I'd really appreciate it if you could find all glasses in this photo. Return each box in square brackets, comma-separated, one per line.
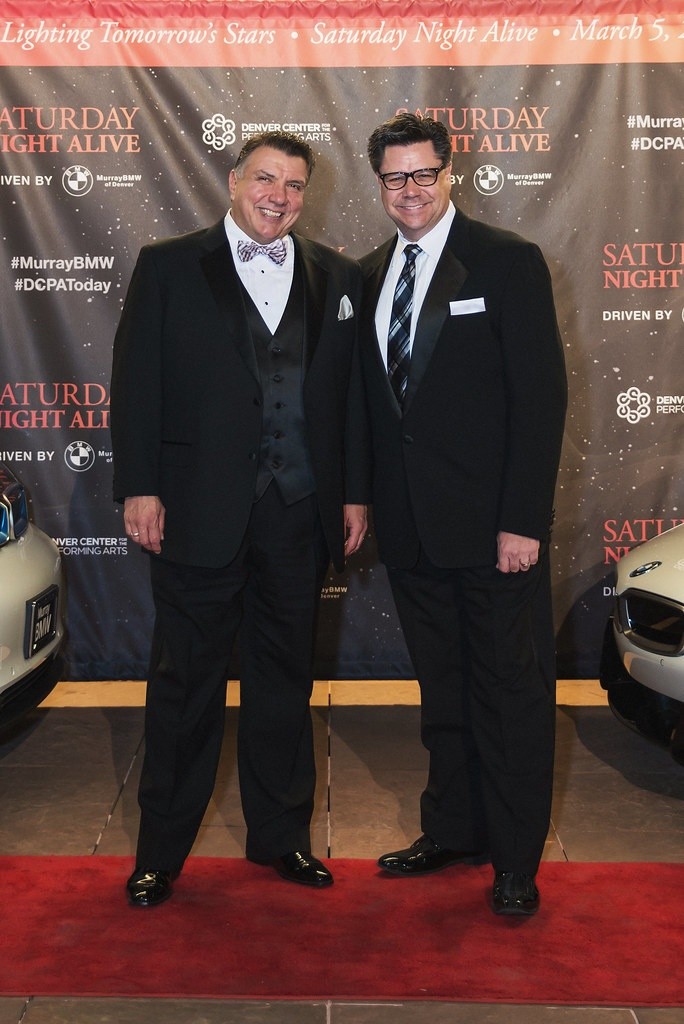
[376, 164, 446, 190]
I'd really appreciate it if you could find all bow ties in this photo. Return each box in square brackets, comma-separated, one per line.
[237, 239, 285, 269]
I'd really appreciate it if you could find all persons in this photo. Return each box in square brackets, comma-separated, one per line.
[355, 113, 568, 914]
[108, 132, 373, 907]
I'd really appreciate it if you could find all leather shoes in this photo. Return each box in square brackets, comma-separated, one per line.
[377, 834, 492, 875]
[491, 870, 540, 915]
[246, 850, 333, 887]
[126, 865, 181, 906]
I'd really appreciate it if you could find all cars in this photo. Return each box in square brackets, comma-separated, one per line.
[602, 520, 684, 704]
[1, 463, 70, 728]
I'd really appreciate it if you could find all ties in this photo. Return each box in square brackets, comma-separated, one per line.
[387, 244, 423, 412]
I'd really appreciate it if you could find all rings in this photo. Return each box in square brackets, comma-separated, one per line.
[131, 532, 139, 536]
[521, 562, 530, 567]
